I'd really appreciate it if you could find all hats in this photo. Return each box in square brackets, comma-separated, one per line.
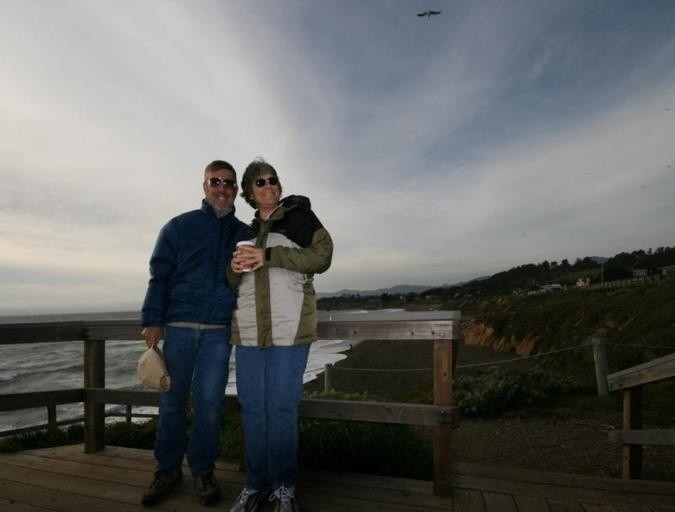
[137, 343, 172, 393]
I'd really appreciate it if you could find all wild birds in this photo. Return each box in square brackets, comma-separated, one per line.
[417, 9, 442, 18]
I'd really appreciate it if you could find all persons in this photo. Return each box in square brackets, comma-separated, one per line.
[226, 163, 330, 511]
[141, 162, 249, 507]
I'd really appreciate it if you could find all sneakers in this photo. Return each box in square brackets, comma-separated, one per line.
[229, 485, 272, 512]
[141, 467, 182, 506]
[194, 465, 222, 506]
[271, 479, 301, 512]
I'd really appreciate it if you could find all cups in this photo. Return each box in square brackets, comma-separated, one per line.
[235, 241, 254, 273]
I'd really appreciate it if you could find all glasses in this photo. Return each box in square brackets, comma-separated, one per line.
[206, 177, 236, 190]
[252, 176, 278, 187]
